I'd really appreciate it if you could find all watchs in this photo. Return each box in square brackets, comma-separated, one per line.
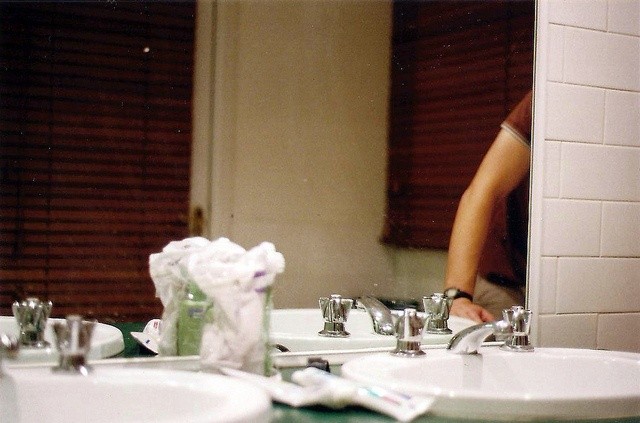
[443, 287, 474, 302]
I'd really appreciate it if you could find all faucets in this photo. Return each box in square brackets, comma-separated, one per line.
[447, 305, 535, 354]
[355, 294, 396, 335]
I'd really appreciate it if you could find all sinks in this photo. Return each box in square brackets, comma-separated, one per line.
[269, 307, 481, 352]
[1, 315, 125, 362]
[341, 343, 640, 420]
[2, 366, 273, 423]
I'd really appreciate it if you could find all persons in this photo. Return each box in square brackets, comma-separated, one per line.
[443, 89, 532, 324]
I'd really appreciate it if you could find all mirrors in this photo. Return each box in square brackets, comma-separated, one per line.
[1, 0, 537, 365]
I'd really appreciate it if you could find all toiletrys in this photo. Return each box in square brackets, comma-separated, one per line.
[242, 285, 272, 376]
